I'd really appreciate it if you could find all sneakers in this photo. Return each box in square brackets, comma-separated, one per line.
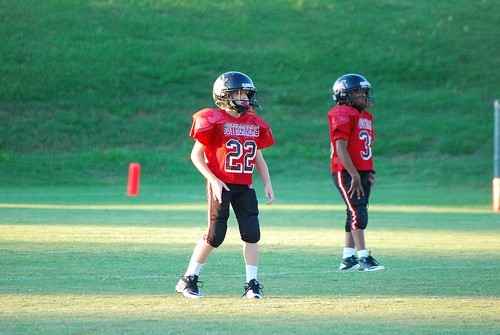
[339, 255, 385, 271]
[176, 274, 203, 299]
[244, 278, 264, 298]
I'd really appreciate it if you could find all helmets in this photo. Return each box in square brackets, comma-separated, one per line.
[213, 71, 258, 114]
[333, 73, 373, 107]
